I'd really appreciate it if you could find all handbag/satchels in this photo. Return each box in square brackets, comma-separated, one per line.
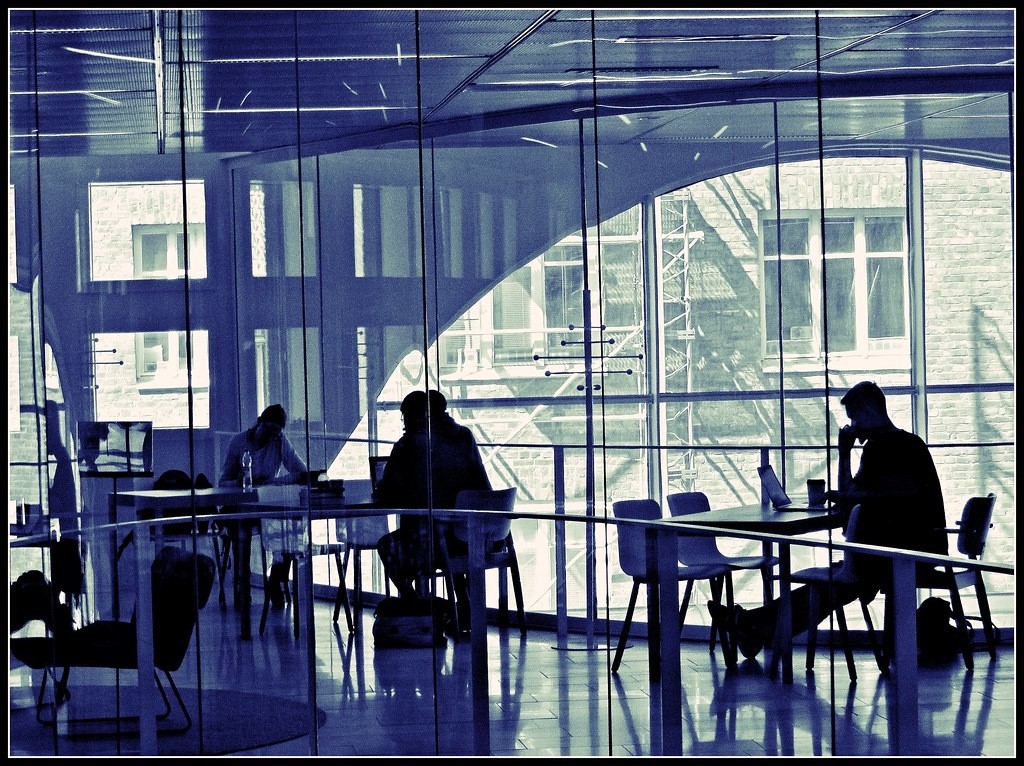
[373, 596, 449, 649]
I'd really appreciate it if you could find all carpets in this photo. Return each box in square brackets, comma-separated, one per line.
[9, 684, 329, 757]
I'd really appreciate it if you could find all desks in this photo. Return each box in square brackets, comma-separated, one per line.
[108, 480, 386, 641]
[644, 495, 853, 687]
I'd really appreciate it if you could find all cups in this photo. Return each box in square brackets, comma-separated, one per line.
[806, 478, 825, 505]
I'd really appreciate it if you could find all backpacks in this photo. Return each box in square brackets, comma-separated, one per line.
[917, 597, 1001, 658]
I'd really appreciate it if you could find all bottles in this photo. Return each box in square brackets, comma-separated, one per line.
[241, 449, 253, 488]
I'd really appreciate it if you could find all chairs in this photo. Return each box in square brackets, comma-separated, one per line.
[9, 453, 529, 743]
[609, 493, 999, 680]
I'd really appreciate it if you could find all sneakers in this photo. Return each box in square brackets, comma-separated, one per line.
[708, 601, 763, 659]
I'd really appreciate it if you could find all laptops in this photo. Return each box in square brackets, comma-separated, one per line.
[369, 455, 390, 494]
[757, 464, 834, 511]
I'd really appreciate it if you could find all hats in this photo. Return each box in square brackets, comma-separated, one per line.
[261, 404, 287, 429]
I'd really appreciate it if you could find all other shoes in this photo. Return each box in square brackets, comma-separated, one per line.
[237, 587, 252, 603]
[268, 577, 284, 604]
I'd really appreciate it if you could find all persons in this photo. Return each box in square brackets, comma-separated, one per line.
[217, 404, 313, 610]
[372, 390, 492, 644]
[708, 381, 948, 659]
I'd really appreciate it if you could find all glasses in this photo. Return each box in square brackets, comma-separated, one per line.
[401, 414, 404, 421]
